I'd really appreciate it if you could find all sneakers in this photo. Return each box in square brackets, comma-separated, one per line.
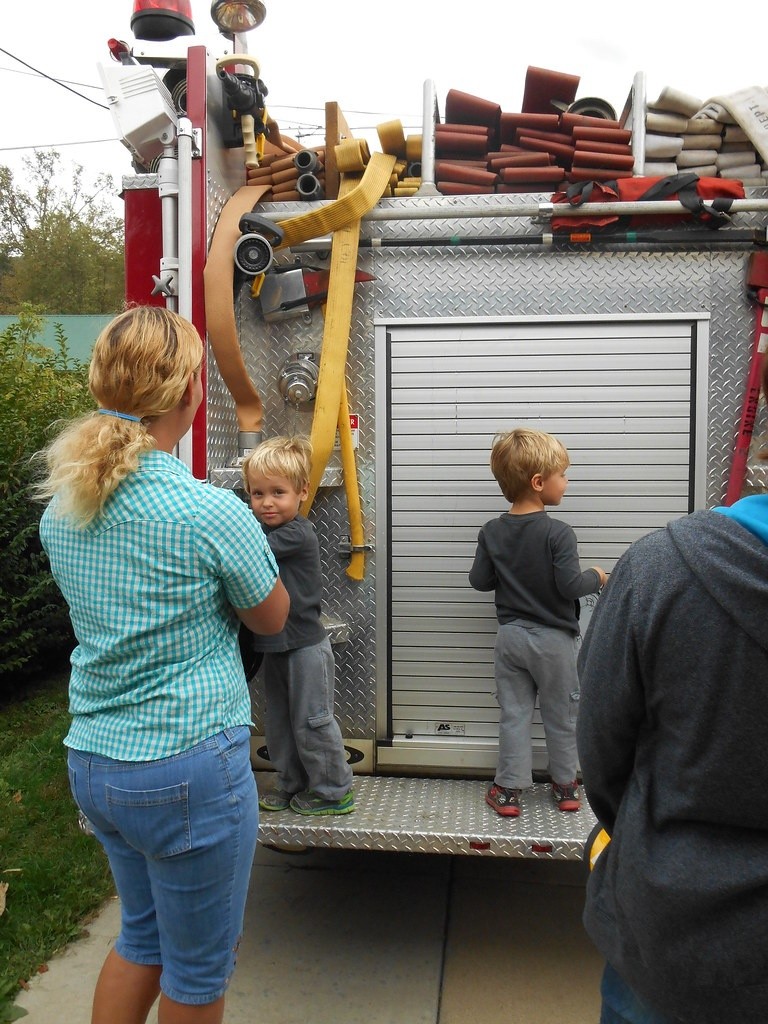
[258, 789, 294, 811]
[485, 783, 522, 817]
[551, 778, 581, 811]
[289, 790, 354, 816]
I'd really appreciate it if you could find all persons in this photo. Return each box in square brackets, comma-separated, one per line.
[31, 306, 292, 1022]
[467, 429, 608, 817]
[574, 493, 768, 1024]
[246, 435, 357, 817]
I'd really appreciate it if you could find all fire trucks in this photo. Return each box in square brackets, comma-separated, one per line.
[104, 0, 767, 874]
